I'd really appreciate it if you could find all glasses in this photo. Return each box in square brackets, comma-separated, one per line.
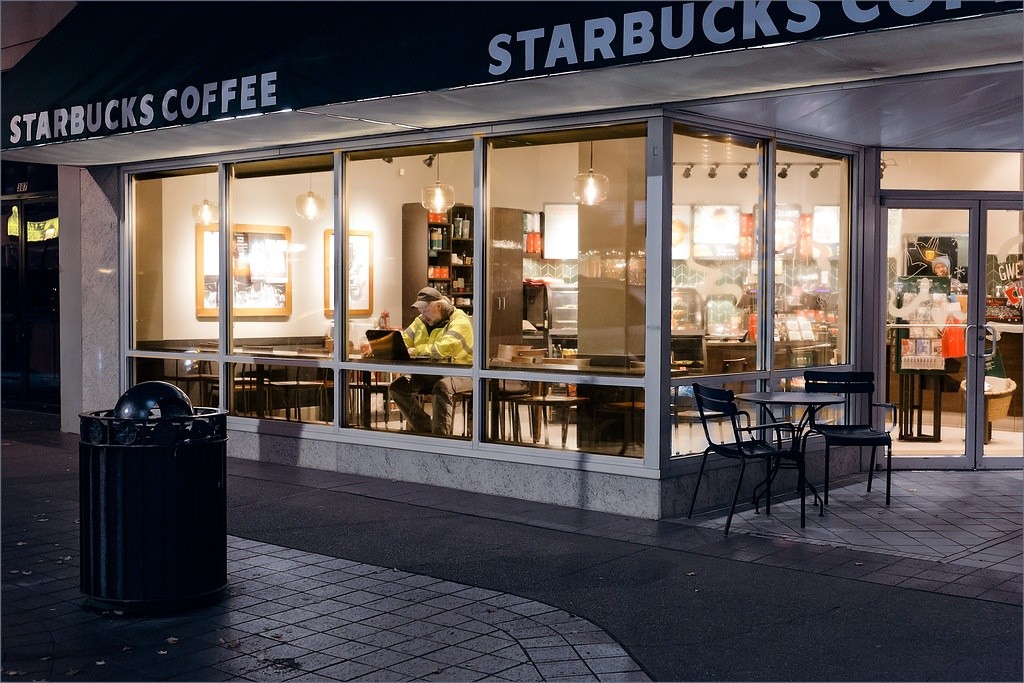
[415, 292, 442, 301]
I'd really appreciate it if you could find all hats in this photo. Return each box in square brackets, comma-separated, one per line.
[932, 258, 950, 274]
[411, 287, 442, 307]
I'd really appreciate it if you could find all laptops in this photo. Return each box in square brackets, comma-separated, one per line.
[365, 330, 431, 360]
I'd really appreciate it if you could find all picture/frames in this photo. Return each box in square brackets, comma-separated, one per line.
[194, 223, 292, 318]
[324, 228, 374, 317]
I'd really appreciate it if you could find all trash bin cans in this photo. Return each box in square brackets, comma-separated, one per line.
[78, 381, 230, 615]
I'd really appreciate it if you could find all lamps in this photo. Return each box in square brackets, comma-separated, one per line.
[296, 173, 324, 220]
[193, 171, 218, 226]
[421, 152, 456, 213]
[571, 139, 609, 208]
[382, 152, 437, 168]
[673, 161, 841, 178]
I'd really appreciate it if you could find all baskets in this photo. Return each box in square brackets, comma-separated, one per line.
[960, 375, 1018, 421]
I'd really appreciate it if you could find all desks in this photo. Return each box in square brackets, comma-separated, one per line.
[733, 391, 846, 519]
[153, 349, 644, 457]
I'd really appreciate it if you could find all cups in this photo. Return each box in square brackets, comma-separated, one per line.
[360, 336, 370, 353]
[464, 298, 471, 306]
[462, 220, 470, 238]
[454, 217, 463, 238]
[452, 297, 455, 305]
[455, 297, 466, 305]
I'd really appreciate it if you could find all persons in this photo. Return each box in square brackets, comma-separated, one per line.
[931, 256, 962, 295]
[389, 286, 473, 436]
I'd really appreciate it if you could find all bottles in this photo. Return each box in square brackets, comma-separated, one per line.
[428, 282, 448, 295]
[562, 348, 578, 359]
[428, 265, 449, 279]
[428, 226, 448, 250]
[551, 343, 563, 358]
[1005, 286, 1009, 297]
[995, 286, 1003, 297]
[523, 227, 541, 253]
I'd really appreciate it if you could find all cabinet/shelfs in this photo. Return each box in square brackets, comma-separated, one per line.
[401, 204, 474, 334]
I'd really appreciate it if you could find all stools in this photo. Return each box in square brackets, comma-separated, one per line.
[895, 355, 946, 441]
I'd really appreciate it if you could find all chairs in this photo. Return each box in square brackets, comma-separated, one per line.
[796, 370, 899, 509]
[149, 342, 748, 457]
[688, 382, 806, 539]
